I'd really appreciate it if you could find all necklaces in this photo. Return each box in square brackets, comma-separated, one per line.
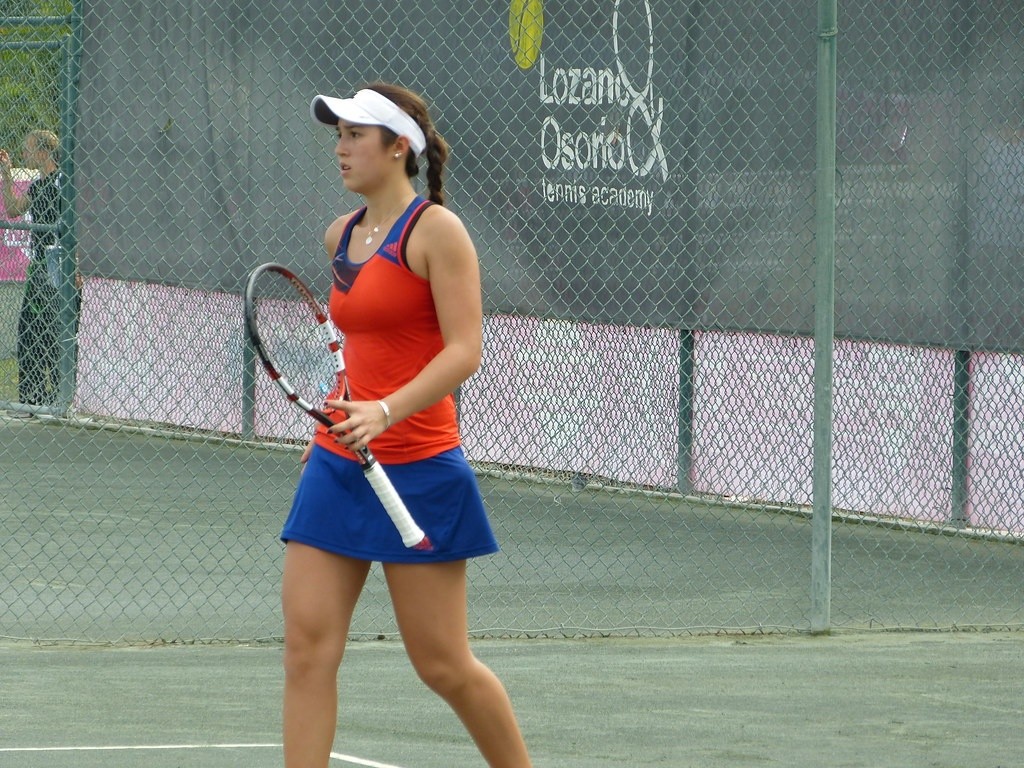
[366, 192, 415, 245]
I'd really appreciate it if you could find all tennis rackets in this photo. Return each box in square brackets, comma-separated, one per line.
[241, 263, 431, 551]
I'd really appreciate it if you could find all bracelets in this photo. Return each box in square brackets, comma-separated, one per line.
[377, 400, 391, 431]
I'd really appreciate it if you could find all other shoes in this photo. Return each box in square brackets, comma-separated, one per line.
[6, 410, 32, 418]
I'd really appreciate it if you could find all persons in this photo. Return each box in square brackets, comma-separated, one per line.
[0, 129, 61, 418]
[279, 81, 532, 768]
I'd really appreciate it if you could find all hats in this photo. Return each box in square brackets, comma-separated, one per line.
[309, 89, 426, 158]
